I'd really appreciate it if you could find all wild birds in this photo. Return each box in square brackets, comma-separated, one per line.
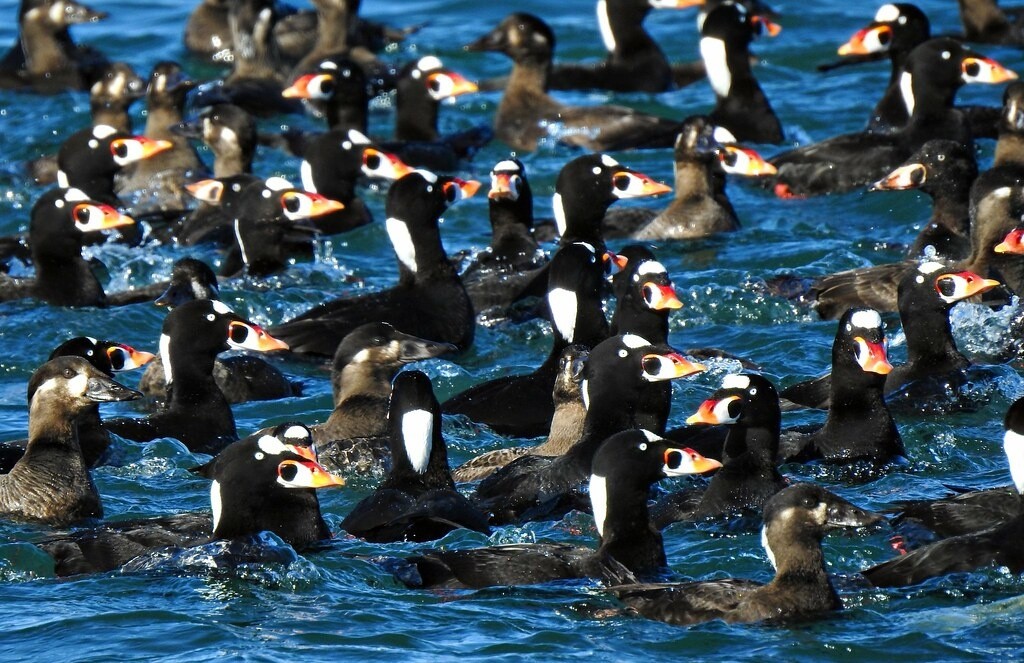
[1, 0, 1024, 632]
